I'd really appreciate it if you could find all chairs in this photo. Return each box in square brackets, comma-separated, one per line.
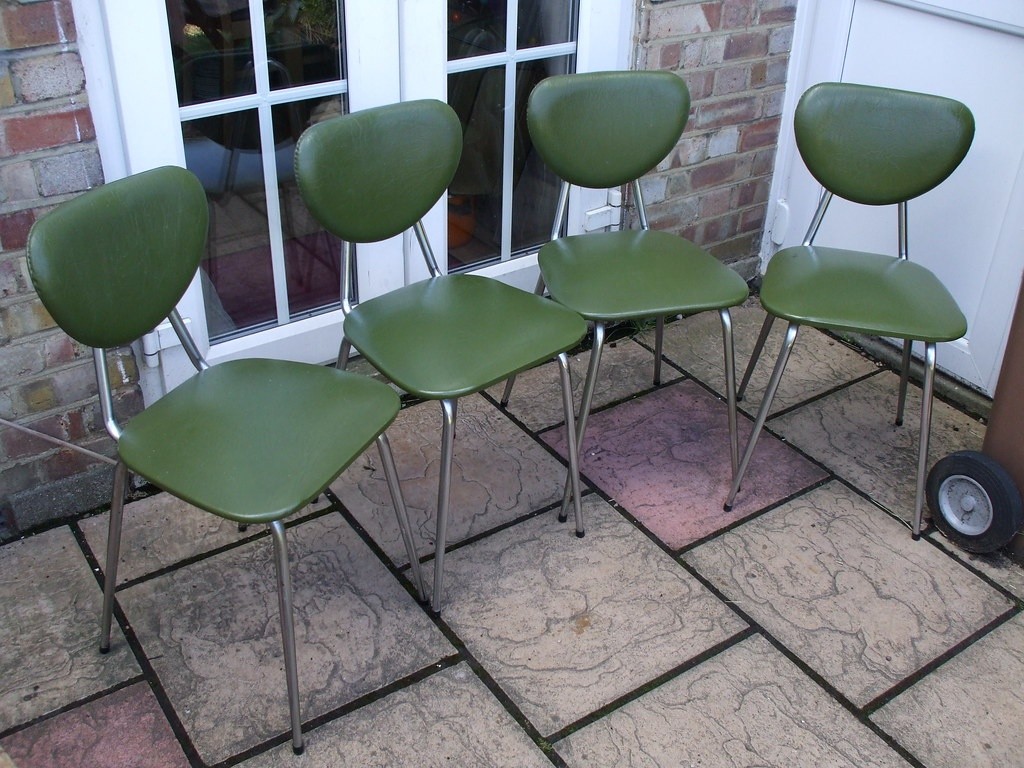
[722, 82, 977, 540]
[27, 167, 431, 755]
[295, 98, 585, 616]
[496, 69, 752, 525]
[176, 0, 546, 311]
